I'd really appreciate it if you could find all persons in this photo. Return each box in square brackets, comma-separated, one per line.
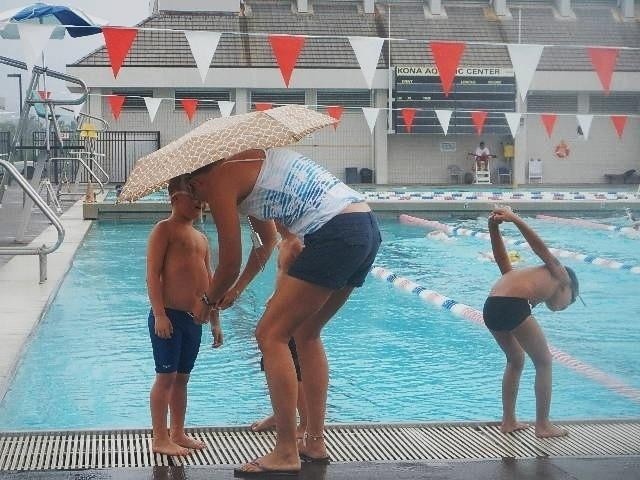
[483, 205, 580, 439]
[184, 145, 383, 474]
[245, 217, 309, 439]
[472, 141, 493, 170]
[144, 173, 224, 456]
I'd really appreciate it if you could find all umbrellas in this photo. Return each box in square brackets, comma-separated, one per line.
[0, 0, 111, 153]
[113, 101, 342, 205]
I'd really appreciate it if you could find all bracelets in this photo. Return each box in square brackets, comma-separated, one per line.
[202, 292, 218, 308]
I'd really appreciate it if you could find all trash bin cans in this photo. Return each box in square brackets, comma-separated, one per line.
[361, 167, 372, 183]
[345, 168, 358, 184]
[448, 163, 465, 184]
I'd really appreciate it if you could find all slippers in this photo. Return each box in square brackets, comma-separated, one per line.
[234, 447, 330, 480]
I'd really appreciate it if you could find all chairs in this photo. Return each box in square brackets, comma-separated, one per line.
[471, 159, 492, 184]
